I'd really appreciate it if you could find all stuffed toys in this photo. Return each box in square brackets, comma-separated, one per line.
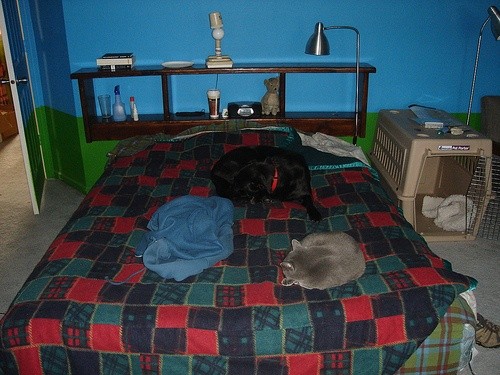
[262, 77, 280, 116]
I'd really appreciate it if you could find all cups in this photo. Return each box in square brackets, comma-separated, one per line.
[99, 96, 112, 118]
[207, 90, 221, 120]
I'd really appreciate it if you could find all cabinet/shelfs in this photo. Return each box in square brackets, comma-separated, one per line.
[70, 62, 376, 139]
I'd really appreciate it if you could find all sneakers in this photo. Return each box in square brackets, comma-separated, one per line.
[476, 312, 500, 348]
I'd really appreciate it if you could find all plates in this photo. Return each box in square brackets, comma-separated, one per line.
[162, 60, 194, 68]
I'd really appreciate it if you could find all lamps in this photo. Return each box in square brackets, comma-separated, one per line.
[204, 11, 233, 69]
[466, 5, 500, 126]
[304, 20, 361, 146]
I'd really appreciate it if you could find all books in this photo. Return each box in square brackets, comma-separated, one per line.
[96, 53, 135, 69]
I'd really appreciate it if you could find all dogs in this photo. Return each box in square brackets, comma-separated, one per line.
[208, 143, 323, 224]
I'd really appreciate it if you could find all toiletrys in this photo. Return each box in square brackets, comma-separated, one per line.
[129, 95, 136, 115]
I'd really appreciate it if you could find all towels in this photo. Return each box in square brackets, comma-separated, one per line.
[422, 192, 478, 234]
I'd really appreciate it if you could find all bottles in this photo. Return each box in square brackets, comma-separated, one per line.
[130, 97, 134, 119]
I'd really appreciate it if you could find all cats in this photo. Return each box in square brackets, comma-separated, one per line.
[279, 230, 367, 292]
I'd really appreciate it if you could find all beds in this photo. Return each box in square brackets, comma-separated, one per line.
[0, 117, 477, 375]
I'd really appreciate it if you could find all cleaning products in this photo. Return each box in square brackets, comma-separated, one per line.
[113, 84, 127, 121]
